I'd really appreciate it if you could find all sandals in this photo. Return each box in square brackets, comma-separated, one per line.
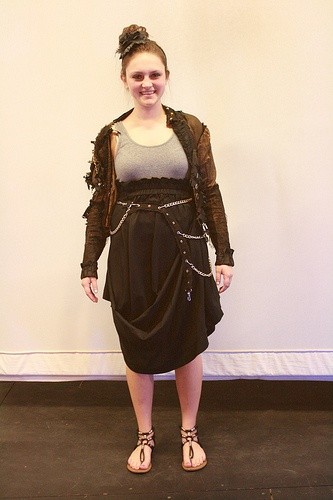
[180, 425, 207, 472]
[126, 426, 156, 475]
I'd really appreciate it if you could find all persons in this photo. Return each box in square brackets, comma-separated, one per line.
[79, 24, 234, 476]
